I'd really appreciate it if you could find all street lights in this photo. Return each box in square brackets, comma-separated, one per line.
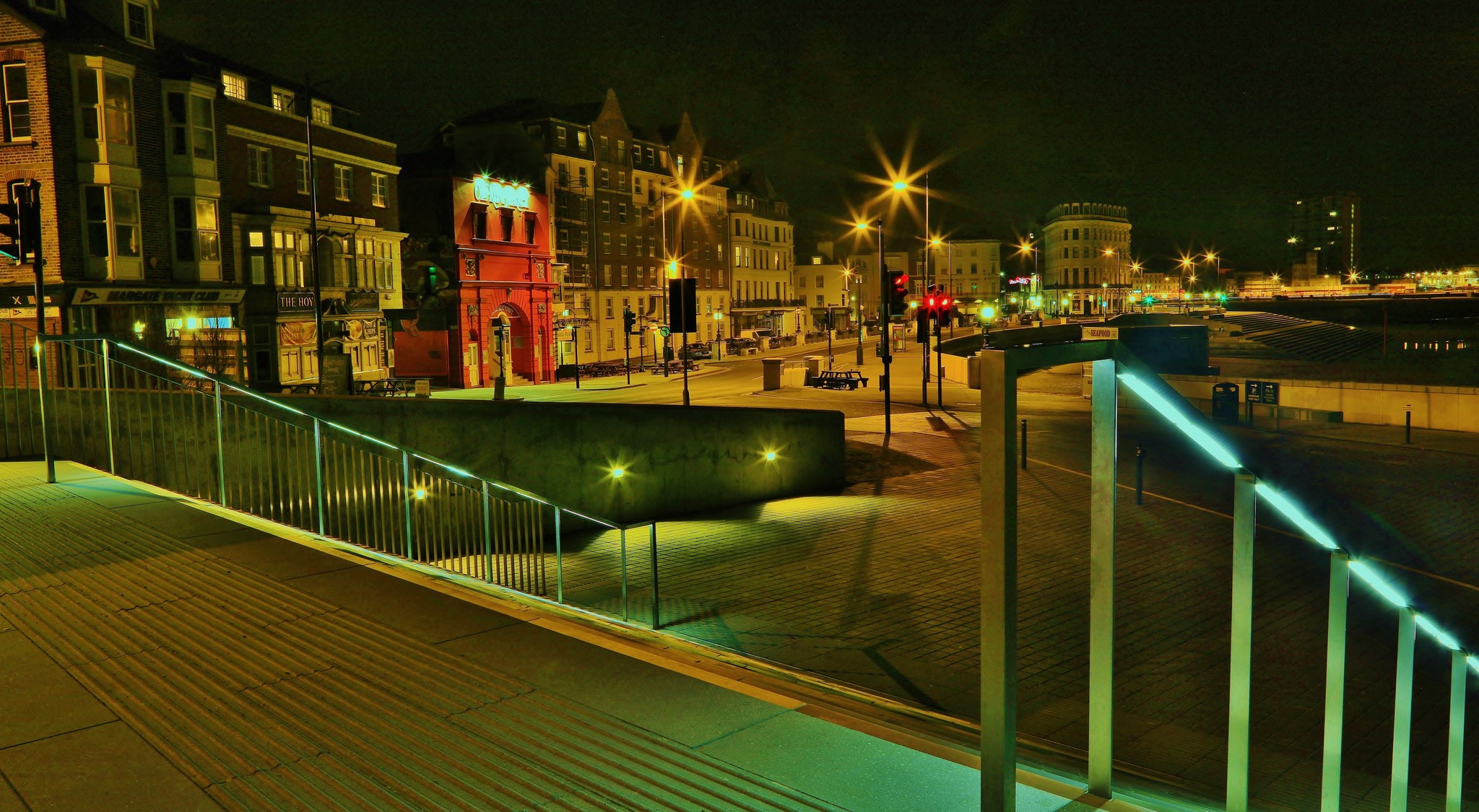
[678, 188, 695, 407]
[1208, 255, 1219, 300]
[713, 308, 723, 361]
[932, 239, 958, 339]
[1106, 249, 1121, 314]
[892, 179, 954, 339]
[1191, 277, 1195, 310]
[795, 304, 803, 333]
[1133, 264, 1143, 311]
[1022, 246, 1038, 314]
[567, 326, 581, 389]
[499, 324, 512, 377]
[1178, 258, 1188, 313]
[1165, 277, 1170, 300]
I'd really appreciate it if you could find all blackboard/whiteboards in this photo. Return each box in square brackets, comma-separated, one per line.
[320, 355, 355, 396]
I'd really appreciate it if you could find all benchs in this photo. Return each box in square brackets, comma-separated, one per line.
[579, 368, 611, 378]
[649, 363, 700, 375]
[812, 376, 869, 390]
[616, 366, 640, 376]
[355, 386, 437, 396]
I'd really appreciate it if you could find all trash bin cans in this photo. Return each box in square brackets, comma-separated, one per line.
[668, 347, 674, 362]
[876, 342, 882, 357]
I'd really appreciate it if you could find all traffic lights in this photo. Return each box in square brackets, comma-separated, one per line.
[0, 198, 29, 267]
[926, 294, 937, 319]
[628, 311, 636, 327]
[888, 269, 909, 316]
[937, 295, 951, 328]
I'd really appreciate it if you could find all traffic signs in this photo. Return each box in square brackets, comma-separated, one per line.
[830, 304, 839, 306]
[1244, 380, 1279, 405]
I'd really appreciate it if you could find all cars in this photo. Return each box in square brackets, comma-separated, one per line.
[983, 315, 1010, 329]
[1020, 314, 1042, 325]
[676, 327, 781, 360]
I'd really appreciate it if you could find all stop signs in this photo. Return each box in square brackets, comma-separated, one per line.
[650, 323, 658, 332]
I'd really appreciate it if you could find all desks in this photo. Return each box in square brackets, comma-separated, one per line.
[816, 371, 862, 390]
[281, 380, 410, 397]
[578, 361, 636, 373]
[654, 359, 697, 375]
[382, 378, 434, 397]
